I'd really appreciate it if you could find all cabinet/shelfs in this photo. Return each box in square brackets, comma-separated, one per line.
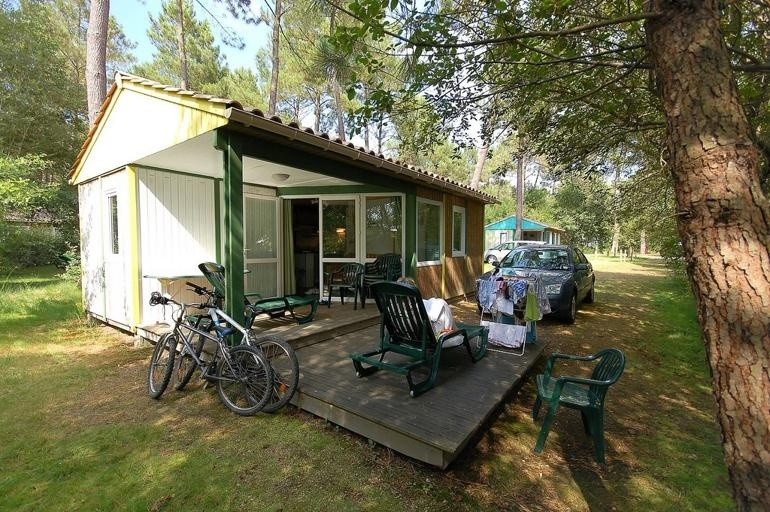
[472, 269, 543, 356]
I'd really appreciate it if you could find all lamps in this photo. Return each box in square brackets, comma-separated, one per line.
[272, 173, 290, 182]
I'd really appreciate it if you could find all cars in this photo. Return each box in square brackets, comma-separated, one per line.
[475, 245, 595, 325]
[485, 240, 547, 266]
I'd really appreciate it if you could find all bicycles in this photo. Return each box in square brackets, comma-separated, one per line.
[146, 280, 299, 416]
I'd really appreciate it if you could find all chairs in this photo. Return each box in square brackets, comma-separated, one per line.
[532, 349, 627, 463]
[326, 251, 402, 310]
[349, 280, 491, 396]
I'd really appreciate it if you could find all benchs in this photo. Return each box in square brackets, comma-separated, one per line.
[185, 261, 320, 333]
